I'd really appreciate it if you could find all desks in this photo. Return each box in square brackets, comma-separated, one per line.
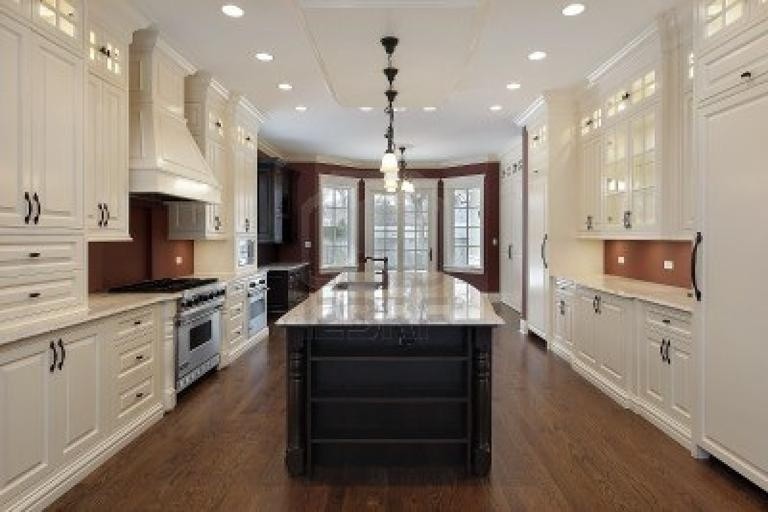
[273, 271, 508, 481]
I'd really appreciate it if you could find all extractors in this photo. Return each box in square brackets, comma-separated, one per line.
[129, 29, 220, 203]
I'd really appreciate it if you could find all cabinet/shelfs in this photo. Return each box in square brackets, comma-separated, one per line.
[691, 1, 768, 494]
[604, 33, 690, 240]
[527, 89, 548, 349]
[0, 0, 165, 512]
[568, 76, 602, 240]
[499, 126, 525, 319]
[195, 97, 258, 274]
[633, 299, 699, 458]
[267, 260, 310, 317]
[165, 293, 174, 374]
[168, 76, 231, 240]
[572, 283, 633, 410]
[548, 275, 572, 369]
[258, 169, 283, 244]
[222, 272, 269, 369]
[174, 280, 221, 373]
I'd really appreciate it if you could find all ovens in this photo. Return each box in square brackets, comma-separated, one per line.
[174, 300, 220, 407]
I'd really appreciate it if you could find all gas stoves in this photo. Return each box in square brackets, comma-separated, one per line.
[108, 277, 226, 317]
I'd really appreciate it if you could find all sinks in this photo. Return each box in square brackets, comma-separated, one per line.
[332, 282, 379, 291]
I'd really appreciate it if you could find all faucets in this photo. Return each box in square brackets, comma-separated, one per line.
[361, 255, 388, 288]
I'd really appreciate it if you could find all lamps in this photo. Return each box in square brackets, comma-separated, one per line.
[377, 34, 415, 194]
